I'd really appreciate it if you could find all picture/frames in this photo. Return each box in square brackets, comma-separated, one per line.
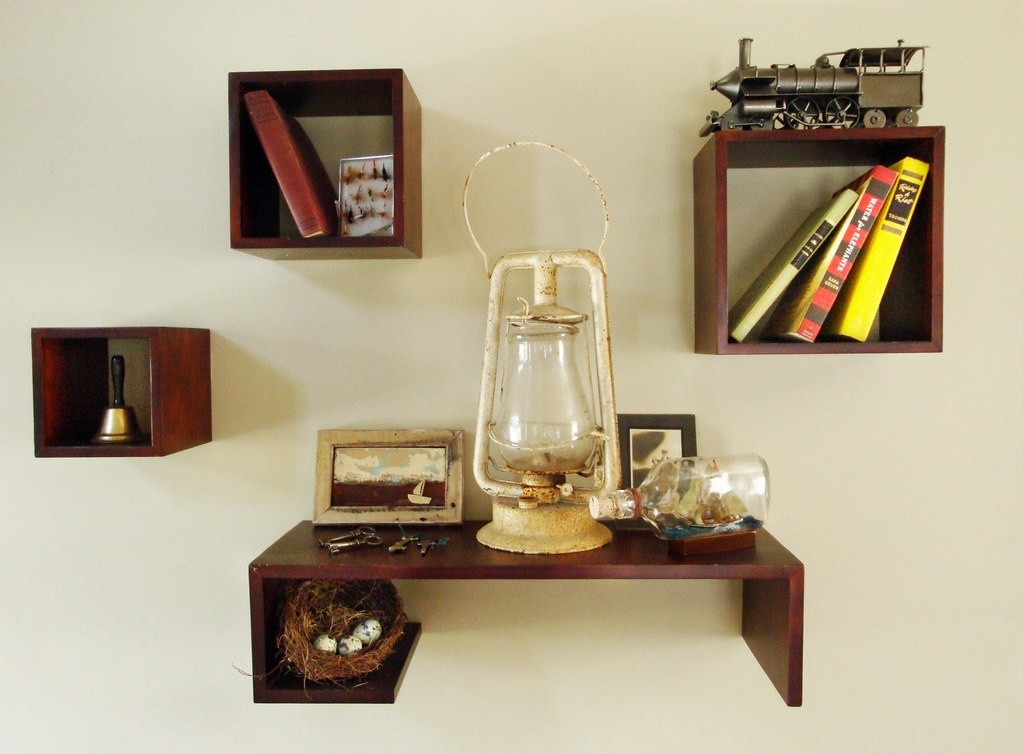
[616, 413, 698, 533]
[314, 430, 463, 527]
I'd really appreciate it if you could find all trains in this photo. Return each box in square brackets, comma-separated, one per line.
[697, 37, 929, 138]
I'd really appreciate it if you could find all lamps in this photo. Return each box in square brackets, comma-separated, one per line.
[464, 141, 623, 556]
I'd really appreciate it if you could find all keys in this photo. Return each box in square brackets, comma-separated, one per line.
[416, 538, 436, 554]
[318, 525, 382, 554]
[388, 531, 421, 552]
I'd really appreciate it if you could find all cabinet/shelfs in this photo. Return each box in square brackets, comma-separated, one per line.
[694, 126, 946, 354]
[226, 70, 422, 259]
[31, 328, 213, 458]
[246, 519, 805, 704]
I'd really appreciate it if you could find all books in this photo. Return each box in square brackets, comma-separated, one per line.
[240, 91, 335, 239]
[729, 154, 931, 344]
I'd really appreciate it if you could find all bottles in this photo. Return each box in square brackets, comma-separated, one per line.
[590, 454, 768, 541]
